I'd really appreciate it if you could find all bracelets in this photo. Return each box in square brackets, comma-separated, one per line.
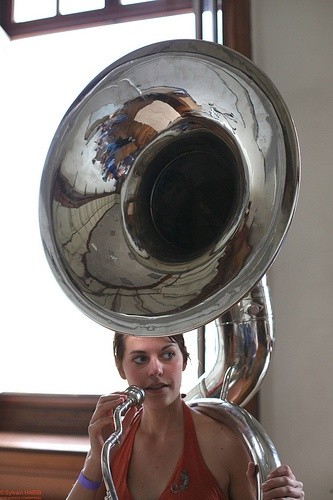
[77, 470, 101, 490]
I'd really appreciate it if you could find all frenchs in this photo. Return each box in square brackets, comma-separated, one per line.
[38, 39, 301, 500]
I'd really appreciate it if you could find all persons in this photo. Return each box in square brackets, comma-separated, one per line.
[66, 331, 304, 500]
